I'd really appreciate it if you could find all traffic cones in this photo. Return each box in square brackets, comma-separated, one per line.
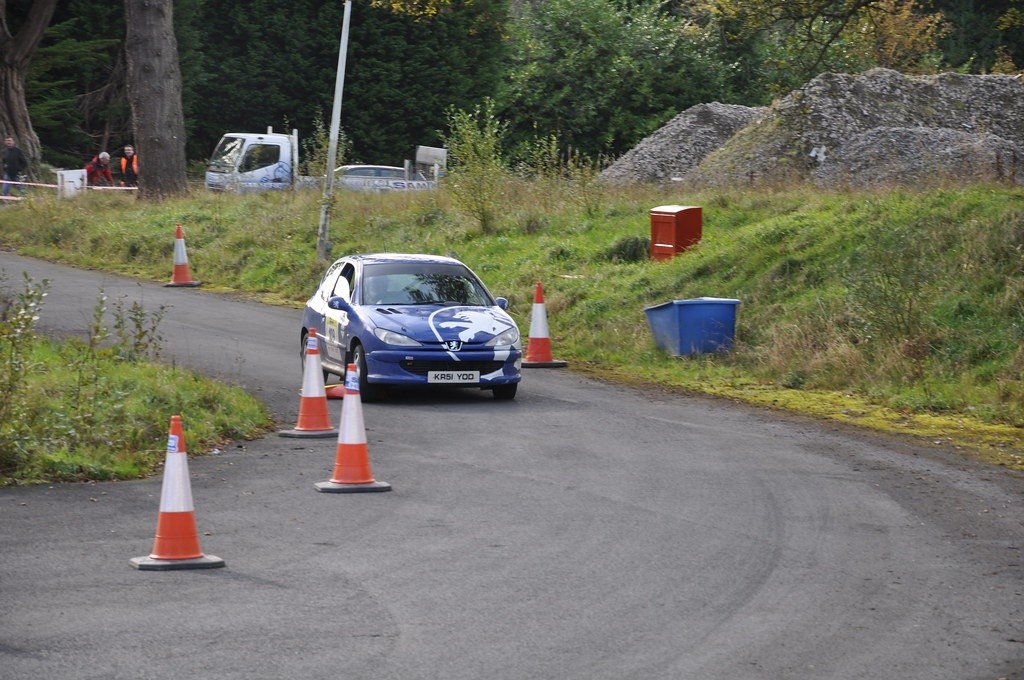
[520, 281, 568, 368]
[324, 384, 345, 399]
[315, 366, 394, 493]
[129, 414, 225, 573]
[278, 326, 340, 438]
[164, 223, 202, 286]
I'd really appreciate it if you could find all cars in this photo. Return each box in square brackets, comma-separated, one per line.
[320, 163, 433, 190]
[299, 252, 521, 404]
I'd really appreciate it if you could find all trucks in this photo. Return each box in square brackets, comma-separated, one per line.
[203, 125, 439, 195]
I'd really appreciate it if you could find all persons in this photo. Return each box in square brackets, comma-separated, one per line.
[119, 144, 138, 187]
[0, 136, 27, 196]
[363, 275, 391, 306]
[84, 152, 115, 190]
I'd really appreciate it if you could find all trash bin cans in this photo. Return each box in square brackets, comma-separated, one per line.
[643, 297, 741, 357]
